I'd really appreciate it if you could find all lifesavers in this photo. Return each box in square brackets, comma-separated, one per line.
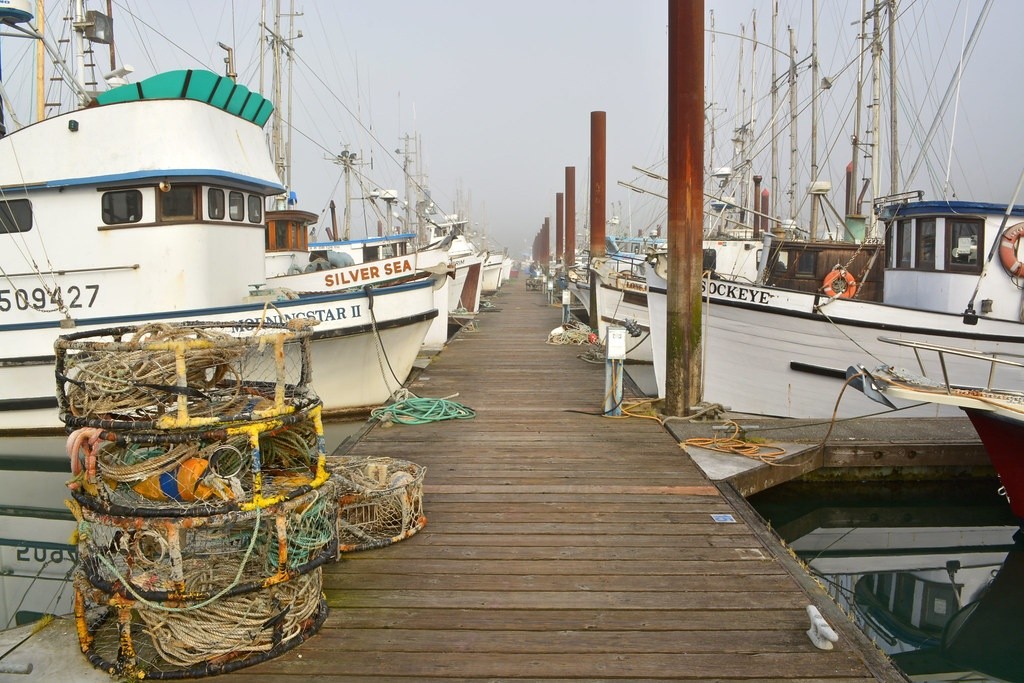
[998, 221, 1024, 279]
[822, 269, 856, 299]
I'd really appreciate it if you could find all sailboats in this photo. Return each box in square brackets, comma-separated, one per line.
[547, 1, 1023, 531]
[0, 0, 522, 636]
